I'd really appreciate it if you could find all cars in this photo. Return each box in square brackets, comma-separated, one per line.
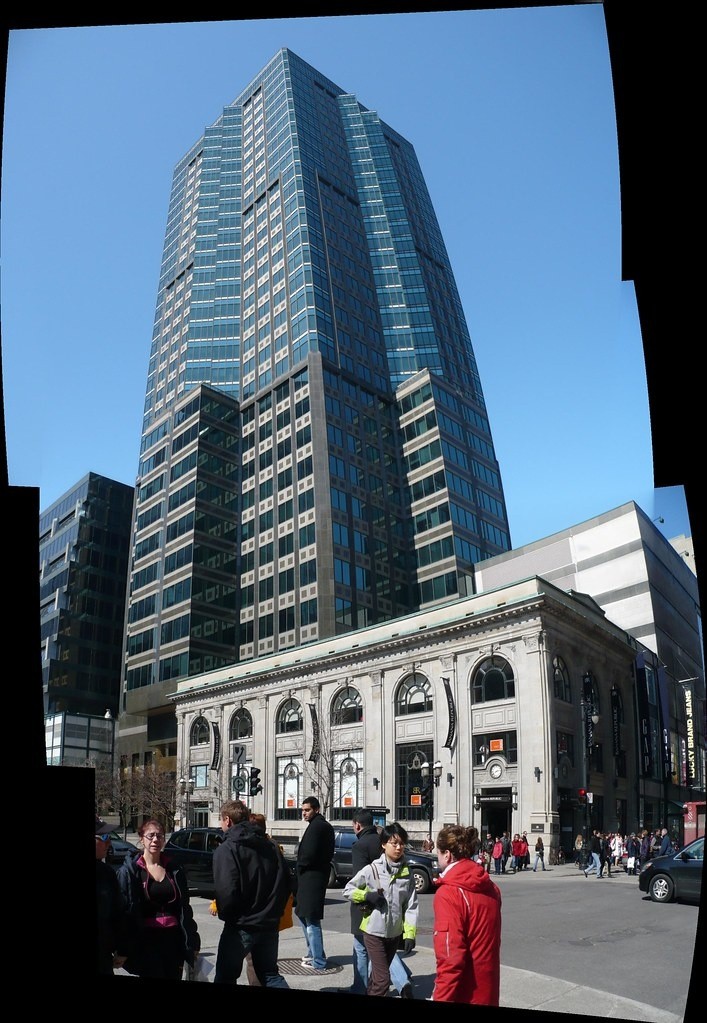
[638, 835, 705, 906]
[95, 821, 140, 866]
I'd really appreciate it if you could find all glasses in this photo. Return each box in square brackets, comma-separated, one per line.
[387, 841, 406, 847]
[95, 834, 111, 841]
[142, 833, 165, 840]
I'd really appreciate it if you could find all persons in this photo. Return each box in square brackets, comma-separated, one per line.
[96, 815, 201, 981]
[574, 835, 583, 866]
[338, 810, 414, 999]
[293, 796, 336, 969]
[208, 800, 292, 989]
[343, 822, 419, 996]
[431, 825, 502, 1006]
[471, 831, 530, 875]
[533, 837, 546, 871]
[584, 829, 672, 878]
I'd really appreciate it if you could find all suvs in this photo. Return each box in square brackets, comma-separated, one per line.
[326, 825, 442, 894]
[159, 825, 229, 892]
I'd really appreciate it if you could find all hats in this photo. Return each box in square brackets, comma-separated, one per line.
[95, 822, 119, 833]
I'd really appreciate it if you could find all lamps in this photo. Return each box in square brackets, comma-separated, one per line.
[213, 787, 217, 797]
[553, 767, 558, 779]
[447, 773, 454, 787]
[311, 782, 315, 792]
[652, 517, 665, 523]
[208, 798, 213, 813]
[678, 551, 689, 557]
[373, 778, 380, 789]
[534, 767, 542, 782]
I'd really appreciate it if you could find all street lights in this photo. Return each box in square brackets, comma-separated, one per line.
[578, 686, 600, 871]
[104, 709, 116, 812]
[420, 760, 444, 853]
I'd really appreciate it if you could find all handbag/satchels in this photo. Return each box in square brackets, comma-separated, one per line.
[627, 856, 635, 869]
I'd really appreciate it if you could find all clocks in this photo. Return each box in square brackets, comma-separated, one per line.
[490, 764, 502, 779]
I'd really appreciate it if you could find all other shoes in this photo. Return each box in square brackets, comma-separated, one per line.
[583, 870, 587, 878]
[401, 984, 412, 999]
[302, 956, 327, 962]
[597, 877, 605, 878]
[301, 961, 326, 970]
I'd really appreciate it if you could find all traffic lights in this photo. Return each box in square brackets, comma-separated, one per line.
[250, 767, 261, 795]
[578, 788, 586, 805]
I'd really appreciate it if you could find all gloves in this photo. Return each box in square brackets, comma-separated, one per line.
[366, 892, 387, 908]
[404, 938, 415, 956]
